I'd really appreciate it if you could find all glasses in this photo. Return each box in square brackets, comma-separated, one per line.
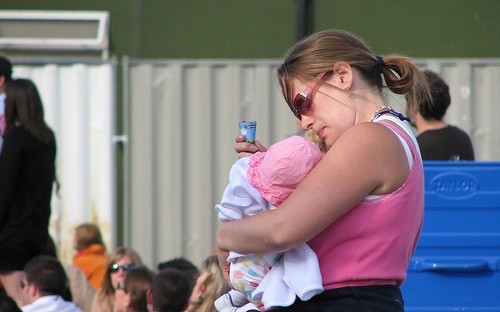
[110, 264, 133, 274]
[117, 283, 128, 293]
[21, 282, 28, 288]
[293, 68, 331, 121]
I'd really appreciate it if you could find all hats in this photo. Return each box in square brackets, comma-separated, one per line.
[247, 134, 324, 206]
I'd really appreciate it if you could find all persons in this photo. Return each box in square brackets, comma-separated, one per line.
[0, 55, 16, 144]
[0, 224, 227, 311]
[213, 136, 323, 311]
[404, 69, 475, 161]
[214, 29, 431, 311]
[1, 79, 56, 255]
